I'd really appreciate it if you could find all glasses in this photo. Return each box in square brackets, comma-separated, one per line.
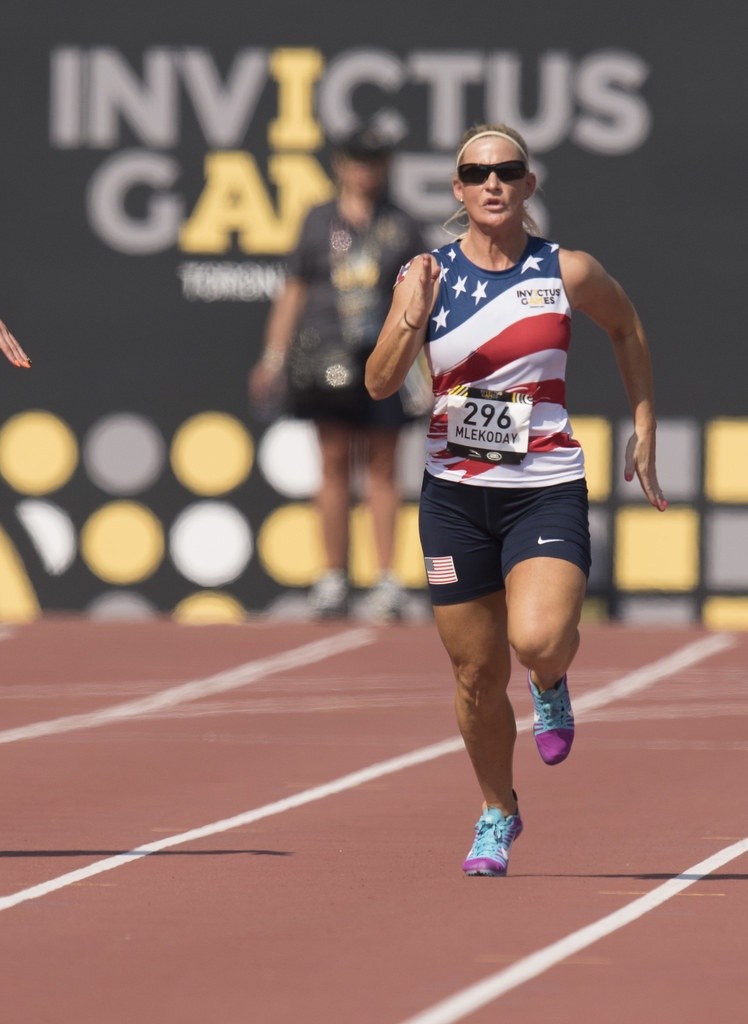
[458, 160, 525, 184]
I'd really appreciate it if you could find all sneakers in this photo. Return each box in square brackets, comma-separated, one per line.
[527, 669, 574, 765]
[462, 789, 522, 876]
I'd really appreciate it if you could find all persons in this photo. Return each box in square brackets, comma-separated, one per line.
[365, 123, 666, 878]
[250, 128, 432, 630]
[0, 319, 33, 369]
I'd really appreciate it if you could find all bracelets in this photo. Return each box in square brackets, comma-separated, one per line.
[404, 311, 421, 329]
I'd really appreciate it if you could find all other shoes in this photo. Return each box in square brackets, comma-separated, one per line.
[373, 586, 410, 625]
[314, 585, 350, 622]
